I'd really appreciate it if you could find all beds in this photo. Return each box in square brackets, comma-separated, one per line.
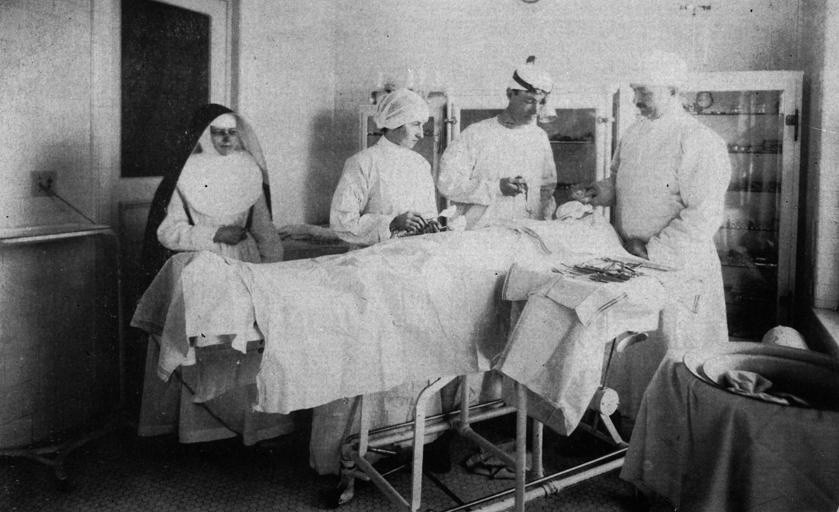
[149, 211, 711, 512]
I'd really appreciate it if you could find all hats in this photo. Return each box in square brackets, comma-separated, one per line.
[508, 65, 553, 93]
[628, 51, 687, 86]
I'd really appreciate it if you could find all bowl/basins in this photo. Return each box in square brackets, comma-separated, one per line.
[680, 337, 836, 419]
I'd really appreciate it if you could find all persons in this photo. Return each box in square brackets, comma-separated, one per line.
[435, 65, 557, 414]
[578, 50, 733, 419]
[306, 90, 442, 477]
[135, 102, 284, 443]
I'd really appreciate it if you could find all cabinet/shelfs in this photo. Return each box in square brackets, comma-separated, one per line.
[608, 68, 803, 342]
[357, 102, 444, 213]
[437, 93, 615, 210]
